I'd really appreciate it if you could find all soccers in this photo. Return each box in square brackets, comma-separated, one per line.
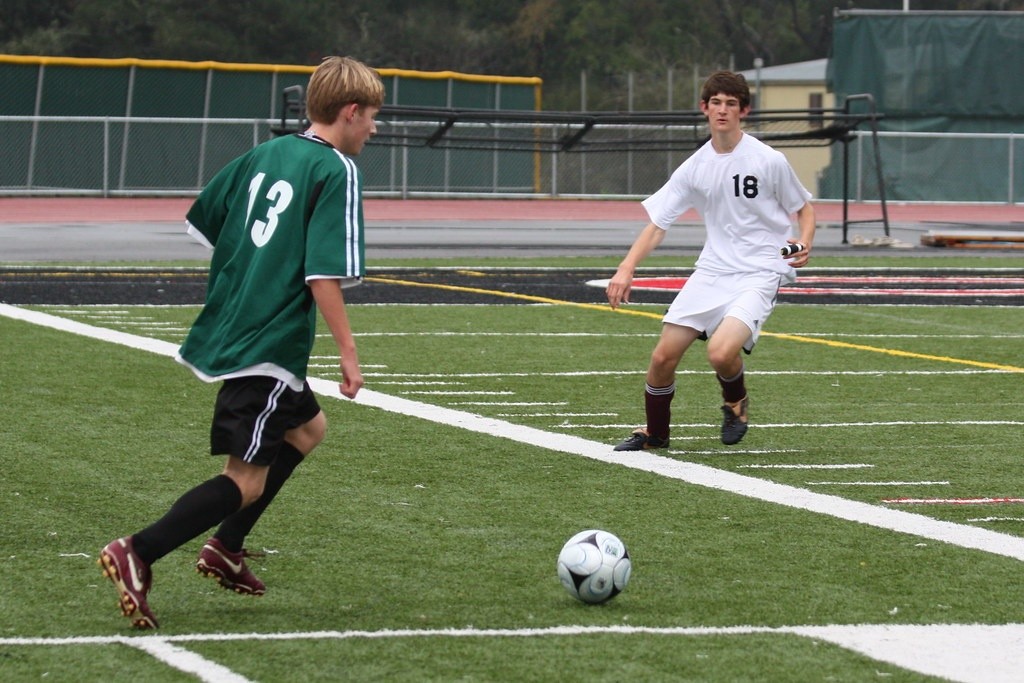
[555, 529, 633, 605]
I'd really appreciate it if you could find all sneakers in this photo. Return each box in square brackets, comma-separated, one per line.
[614, 430, 670, 452]
[97, 535, 160, 631]
[197, 537, 268, 597]
[721, 394, 749, 446]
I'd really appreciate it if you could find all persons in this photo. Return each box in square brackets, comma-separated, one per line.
[97, 56, 386, 631]
[605, 70, 816, 452]
